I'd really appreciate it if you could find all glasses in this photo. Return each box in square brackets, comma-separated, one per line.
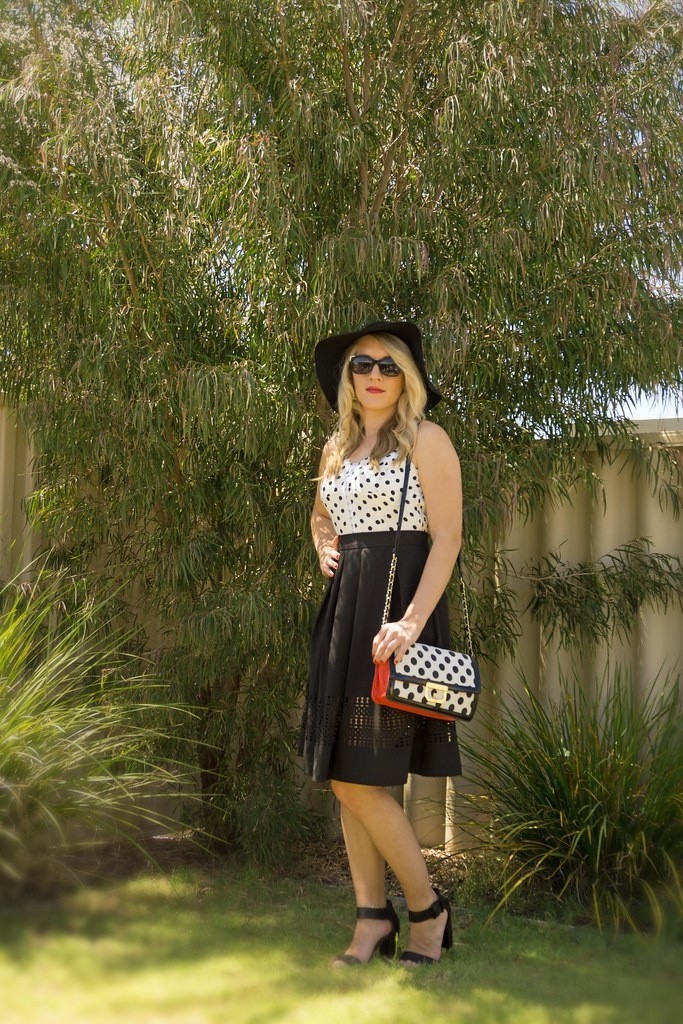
[349, 355, 402, 376]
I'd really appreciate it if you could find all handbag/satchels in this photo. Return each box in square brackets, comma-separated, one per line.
[371, 641, 481, 721]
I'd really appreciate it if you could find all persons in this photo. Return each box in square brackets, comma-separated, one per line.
[295, 320, 462, 967]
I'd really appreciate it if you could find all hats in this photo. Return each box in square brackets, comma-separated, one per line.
[315, 321, 443, 414]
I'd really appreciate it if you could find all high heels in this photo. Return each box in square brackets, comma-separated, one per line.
[336, 900, 400, 967]
[398, 888, 452, 966]
